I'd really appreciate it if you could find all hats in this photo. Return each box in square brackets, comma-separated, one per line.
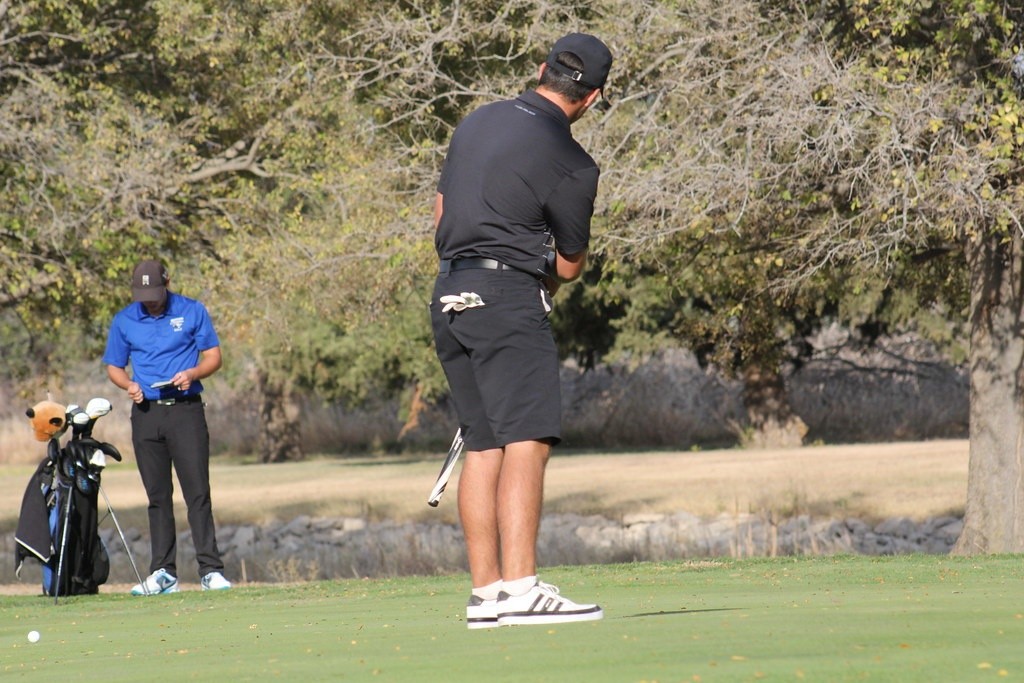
[543, 33, 614, 99]
[131, 260, 171, 302]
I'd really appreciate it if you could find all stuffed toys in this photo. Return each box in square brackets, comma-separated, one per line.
[26, 400, 66, 442]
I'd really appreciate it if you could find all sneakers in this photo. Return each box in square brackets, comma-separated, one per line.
[465, 591, 500, 627]
[201, 570, 232, 590]
[132, 568, 180, 597]
[489, 578, 605, 626]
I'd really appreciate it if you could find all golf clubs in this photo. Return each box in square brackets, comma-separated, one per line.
[427, 426, 464, 507]
[46, 397, 123, 495]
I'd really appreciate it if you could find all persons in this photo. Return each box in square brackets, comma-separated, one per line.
[429, 32, 612, 627]
[101, 259, 232, 596]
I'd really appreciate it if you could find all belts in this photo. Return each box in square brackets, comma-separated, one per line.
[148, 395, 204, 406]
[436, 254, 515, 273]
